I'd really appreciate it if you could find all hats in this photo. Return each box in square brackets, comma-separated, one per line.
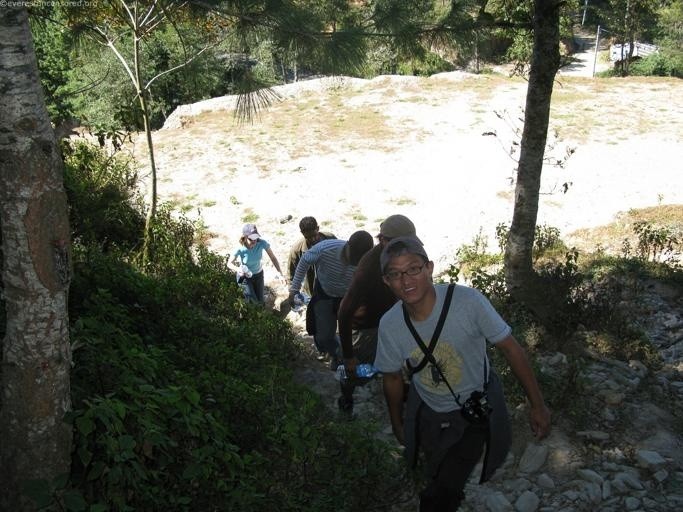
[380, 215, 424, 246]
[380, 237, 428, 274]
[242, 224, 261, 240]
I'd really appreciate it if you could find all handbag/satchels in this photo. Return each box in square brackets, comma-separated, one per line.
[236, 273, 248, 285]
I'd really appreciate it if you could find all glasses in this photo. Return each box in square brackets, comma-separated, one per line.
[386, 261, 426, 280]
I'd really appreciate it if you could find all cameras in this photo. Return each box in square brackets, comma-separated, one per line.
[460, 391, 494, 425]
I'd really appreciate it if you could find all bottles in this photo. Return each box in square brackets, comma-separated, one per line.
[240, 265, 253, 279]
[337, 363, 379, 378]
[291, 293, 304, 312]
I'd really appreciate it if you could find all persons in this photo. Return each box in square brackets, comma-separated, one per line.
[284, 215, 339, 361]
[368, 233, 554, 511]
[333, 211, 433, 411]
[284, 229, 374, 371]
[224, 222, 287, 313]
